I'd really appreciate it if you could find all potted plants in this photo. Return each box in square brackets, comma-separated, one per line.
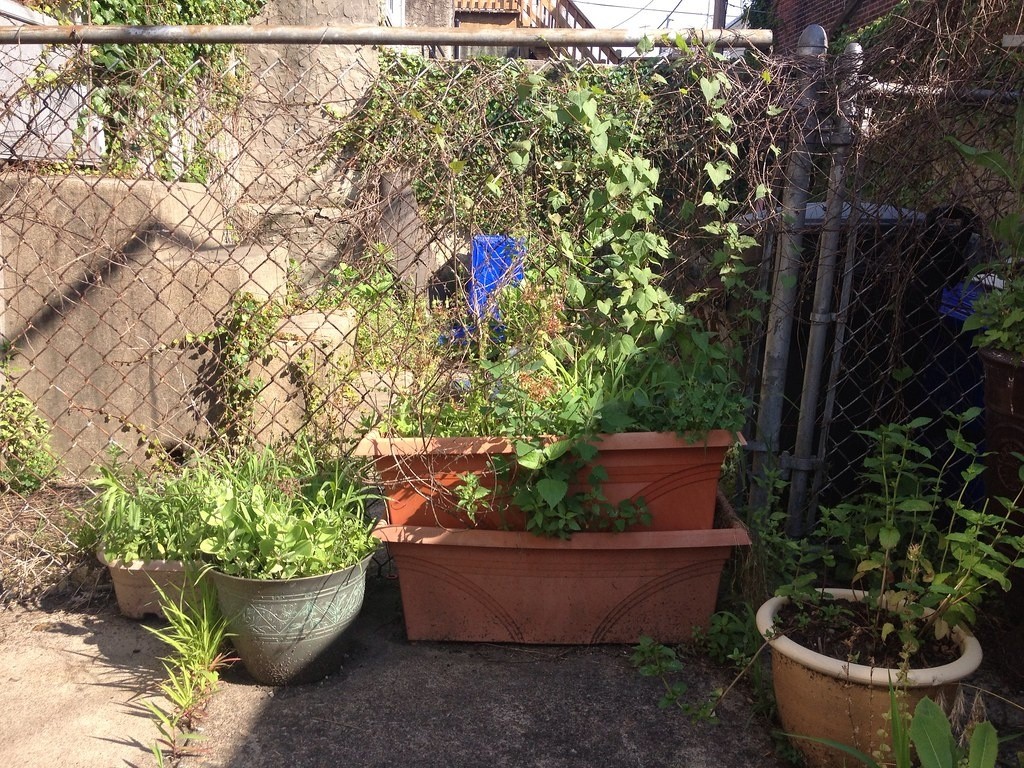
[756, 407, 1024, 768]
[198, 482, 376, 687]
[85, 461, 215, 619]
[373, 348, 751, 530]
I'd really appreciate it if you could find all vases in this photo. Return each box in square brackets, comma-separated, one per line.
[371, 487, 752, 644]
